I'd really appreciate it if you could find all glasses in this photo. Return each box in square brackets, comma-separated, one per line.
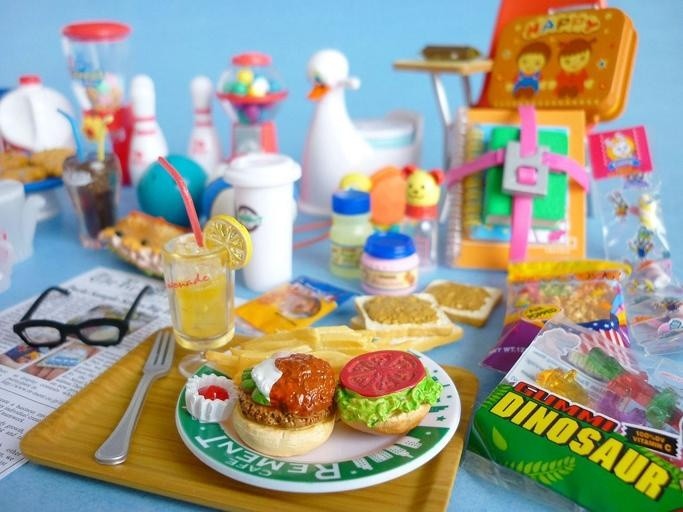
[12, 285, 150, 348]
